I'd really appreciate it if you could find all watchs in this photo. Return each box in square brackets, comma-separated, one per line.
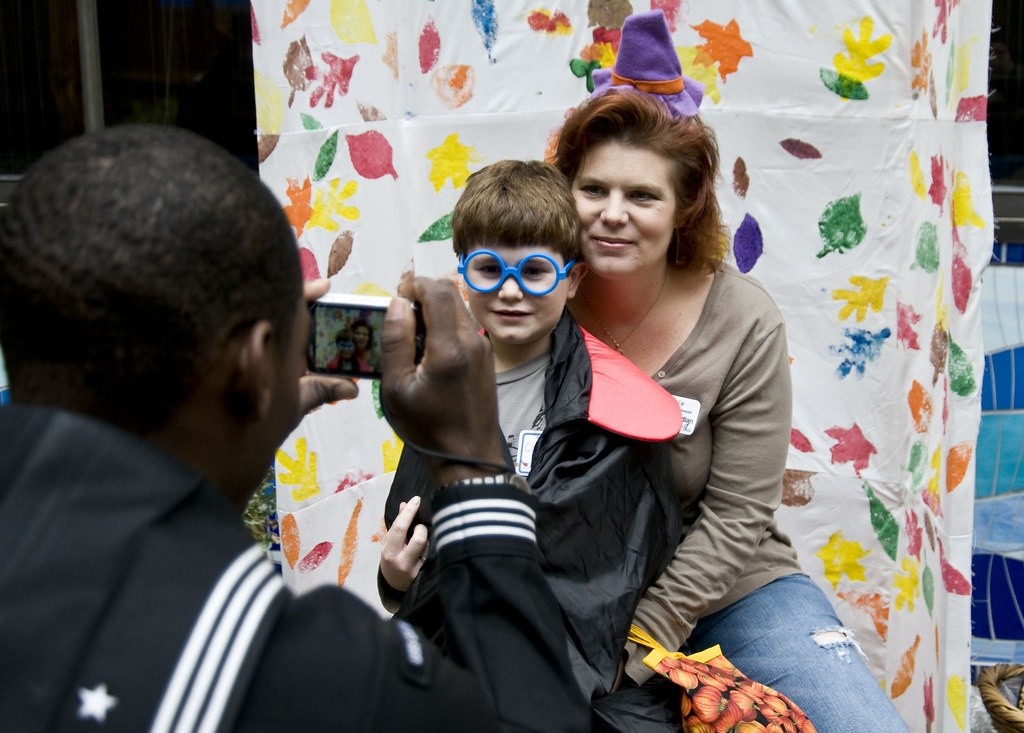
[424, 473, 532, 499]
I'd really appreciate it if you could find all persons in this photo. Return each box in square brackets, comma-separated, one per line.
[489, 85, 921, 733]
[0, 125, 596, 733]
[343, 320, 380, 371]
[374, 159, 690, 732]
[323, 328, 372, 371]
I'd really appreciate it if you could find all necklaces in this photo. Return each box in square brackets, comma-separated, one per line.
[577, 264, 671, 358]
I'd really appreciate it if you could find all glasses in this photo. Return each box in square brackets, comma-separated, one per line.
[457, 250, 577, 297]
[338, 342, 354, 350]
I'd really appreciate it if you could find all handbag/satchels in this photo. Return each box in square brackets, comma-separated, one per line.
[625, 623, 819, 733]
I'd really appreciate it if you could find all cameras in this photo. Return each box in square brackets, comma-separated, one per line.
[303, 294, 428, 380]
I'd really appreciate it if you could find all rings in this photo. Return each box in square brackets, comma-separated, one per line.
[418, 554, 426, 564]
